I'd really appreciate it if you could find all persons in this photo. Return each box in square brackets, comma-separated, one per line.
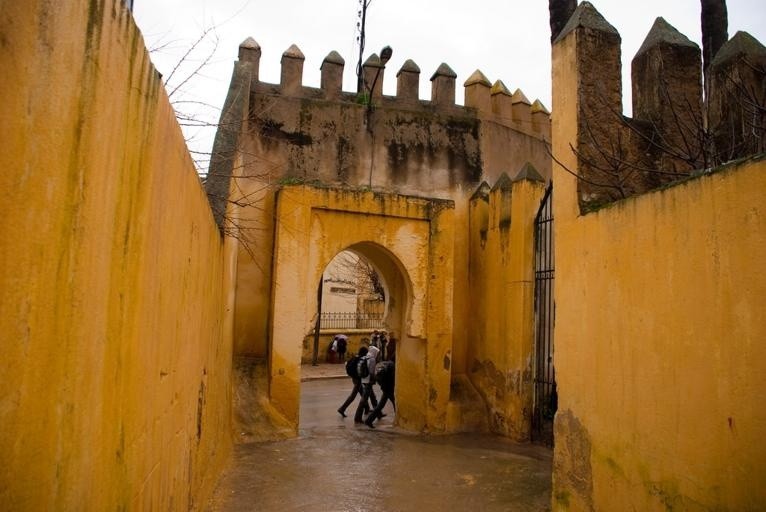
[366, 351, 395, 428]
[330, 337, 338, 364]
[354, 345, 388, 425]
[337, 346, 374, 418]
[337, 338, 347, 363]
[370, 330, 396, 361]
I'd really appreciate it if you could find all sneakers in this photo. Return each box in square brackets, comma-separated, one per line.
[337, 409, 386, 429]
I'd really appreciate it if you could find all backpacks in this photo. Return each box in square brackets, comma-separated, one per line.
[359, 358, 370, 379]
[346, 355, 363, 378]
[375, 360, 393, 386]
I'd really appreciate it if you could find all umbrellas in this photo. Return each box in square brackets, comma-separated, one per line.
[335, 334, 347, 340]
